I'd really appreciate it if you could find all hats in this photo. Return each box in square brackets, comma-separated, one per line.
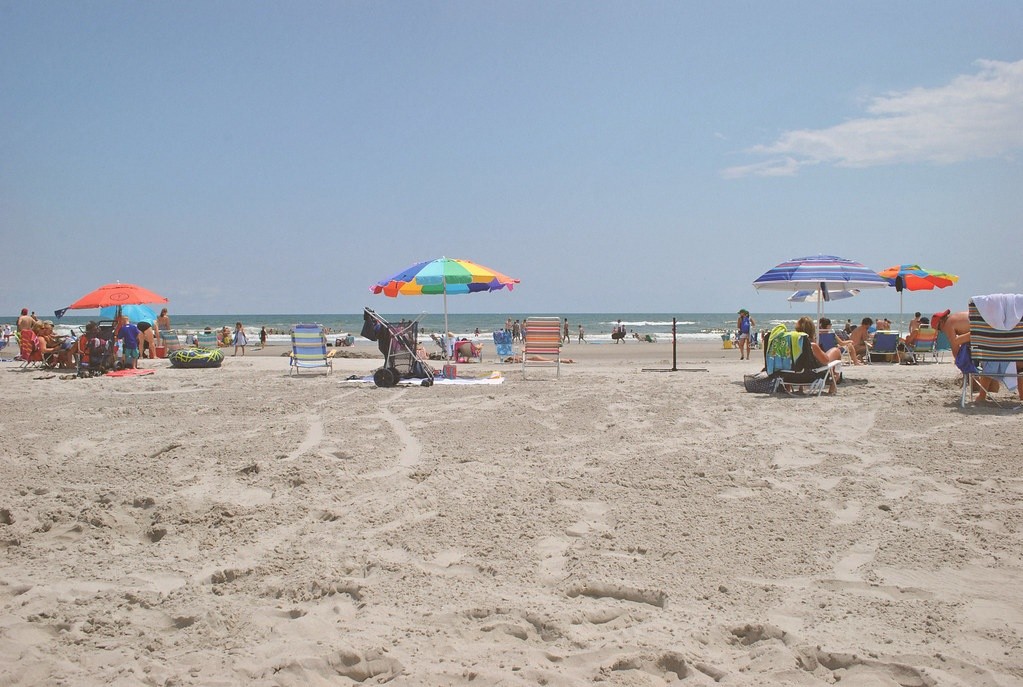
[917, 317, 930, 324]
[931, 309, 950, 328]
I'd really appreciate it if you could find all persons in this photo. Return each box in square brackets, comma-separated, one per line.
[506, 355, 575, 363]
[736, 309, 864, 395]
[269, 329, 286, 335]
[323, 327, 342, 335]
[500, 317, 526, 344]
[931, 308, 1023, 401]
[231, 323, 249, 357]
[17, 308, 76, 368]
[562, 318, 587, 344]
[462, 338, 484, 353]
[259, 325, 268, 347]
[0, 323, 10, 347]
[474, 327, 480, 337]
[157, 308, 170, 330]
[342, 333, 355, 347]
[72, 316, 140, 369]
[194, 327, 224, 346]
[849, 312, 929, 364]
[612, 319, 657, 344]
[222, 326, 232, 347]
[136, 319, 160, 358]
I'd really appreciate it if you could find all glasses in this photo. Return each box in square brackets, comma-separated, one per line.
[937, 321, 941, 331]
[46, 324, 55, 328]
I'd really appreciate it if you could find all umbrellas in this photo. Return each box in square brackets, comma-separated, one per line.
[368, 257, 521, 364]
[752, 253, 959, 345]
[97, 304, 159, 326]
[68, 279, 169, 317]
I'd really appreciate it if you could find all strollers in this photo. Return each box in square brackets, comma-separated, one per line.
[363, 305, 436, 385]
[428, 334, 446, 360]
[76, 319, 123, 377]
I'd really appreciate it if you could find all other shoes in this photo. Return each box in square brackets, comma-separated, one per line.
[33, 370, 102, 380]
[740, 357, 744, 360]
[746, 358, 749, 360]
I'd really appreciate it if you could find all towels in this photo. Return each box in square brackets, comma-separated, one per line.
[19, 328, 40, 364]
[970, 294, 1023, 391]
[765, 324, 803, 376]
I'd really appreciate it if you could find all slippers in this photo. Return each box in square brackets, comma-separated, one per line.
[347, 375, 360, 380]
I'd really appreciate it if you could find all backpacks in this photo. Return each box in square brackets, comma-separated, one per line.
[741, 316, 750, 333]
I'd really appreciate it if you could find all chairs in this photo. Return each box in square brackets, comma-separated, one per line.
[955, 293, 1023, 410]
[335, 336, 355, 348]
[160, 329, 223, 351]
[493, 331, 521, 364]
[431, 335, 483, 364]
[289, 323, 336, 377]
[521, 317, 563, 381]
[762, 323, 952, 397]
[721, 334, 734, 349]
[14, 329, 60, 370]
